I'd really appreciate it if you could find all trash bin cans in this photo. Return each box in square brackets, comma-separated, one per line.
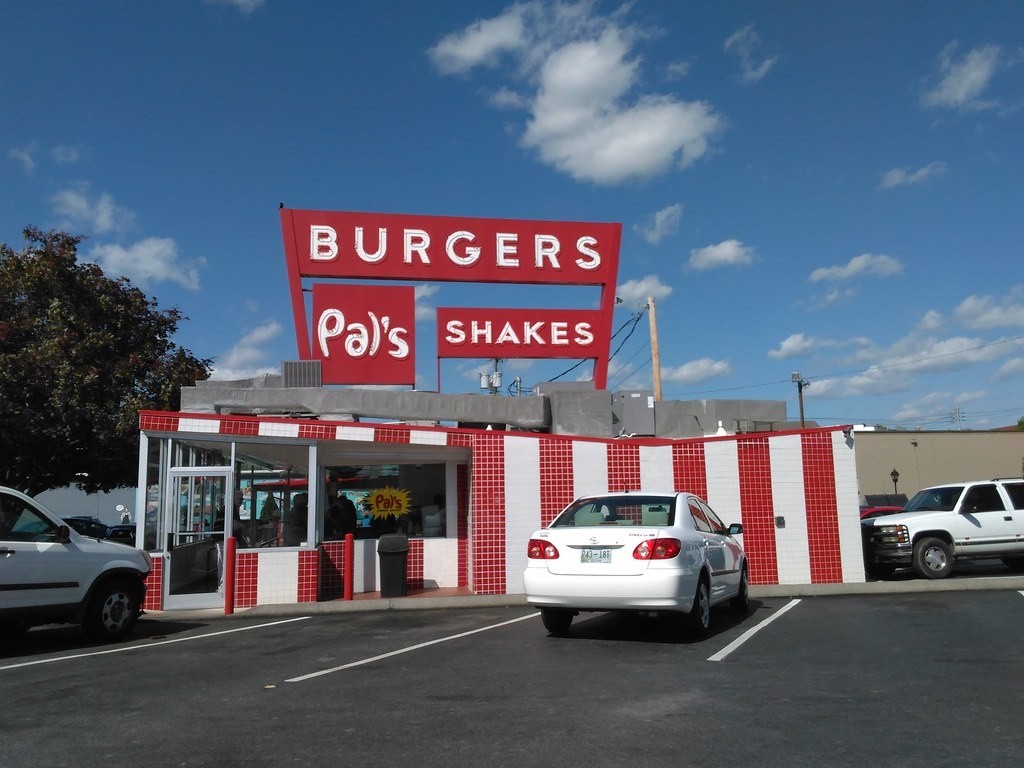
[377, 534, 409, 597]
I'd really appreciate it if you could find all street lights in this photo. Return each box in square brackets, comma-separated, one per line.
[791, 371, 805, 428]
[890, 469, 899, 494]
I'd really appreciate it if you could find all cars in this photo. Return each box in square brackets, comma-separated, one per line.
[0, 486, 153, 649]
[859, 505, 904, 520]
[18, 516, 156, 551]
[524, 491, 749, 642]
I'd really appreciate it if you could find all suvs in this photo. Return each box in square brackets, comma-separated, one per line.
[860, 478, 1024, 580]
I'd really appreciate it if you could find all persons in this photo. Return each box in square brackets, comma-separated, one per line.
[369, 514, 395, 537]
[121, 507, 131, 524]
[362, 515, 371, 526]
[215, 490, 243, 540]
[286, 494, 308, 545]
[328, 495, 356, 539]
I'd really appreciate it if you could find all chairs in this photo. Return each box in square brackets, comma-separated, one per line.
[643, 512, 668, 525]
[575, 512, 604, 526]
